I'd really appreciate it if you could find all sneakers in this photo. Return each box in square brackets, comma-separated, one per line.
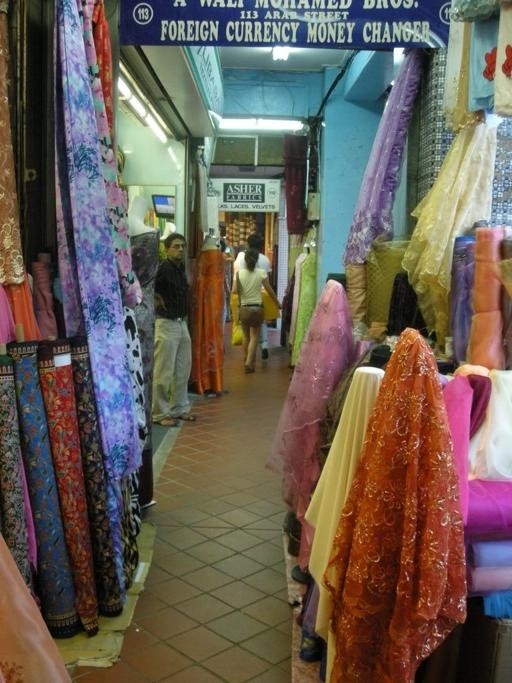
[243, 348, 269, 373]
[154, 413, 196, 426]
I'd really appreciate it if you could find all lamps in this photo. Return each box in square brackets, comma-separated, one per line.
[117, 68, 168, 144]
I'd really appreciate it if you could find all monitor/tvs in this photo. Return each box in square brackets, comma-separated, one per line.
[152, 194, 175, 219]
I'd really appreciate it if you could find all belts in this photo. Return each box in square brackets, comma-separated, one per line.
[242, 303, 264, 308]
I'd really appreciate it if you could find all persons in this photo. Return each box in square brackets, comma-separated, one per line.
[152, 232, 197, 427]
[286, 244, 308, 347]
[289, 241, 318, 369]
[194, 234, 228, 394]
[231, 234, 272, 359]
[235, 246, 284, 373]
